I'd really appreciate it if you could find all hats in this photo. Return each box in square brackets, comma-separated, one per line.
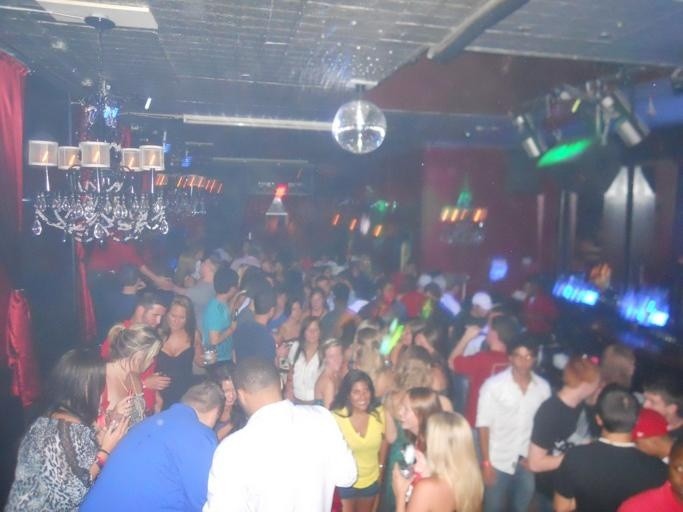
[632, 409, 668, 441]
[472, 292, 493, 311]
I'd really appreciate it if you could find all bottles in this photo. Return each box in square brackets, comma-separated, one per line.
[464, 325, 486, 338]
[396, 444, 416, 480]
[232, 308, 238, 332]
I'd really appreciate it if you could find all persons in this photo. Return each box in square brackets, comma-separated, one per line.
[2, 219, 682, 511]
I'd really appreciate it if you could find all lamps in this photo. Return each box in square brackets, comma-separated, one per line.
[509, 62, 654, 160]
[27, 15, 170, 243]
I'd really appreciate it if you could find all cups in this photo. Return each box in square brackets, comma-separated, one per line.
[202, 342, 216, 368]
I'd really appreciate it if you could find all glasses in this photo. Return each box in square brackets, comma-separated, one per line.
[515, 354, 532, 360]
